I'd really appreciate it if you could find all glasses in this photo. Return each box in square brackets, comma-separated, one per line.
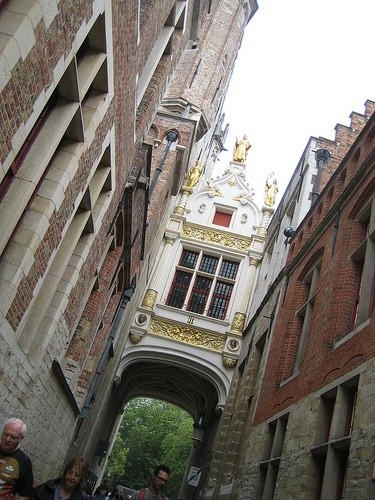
[155, 474, 168, 484]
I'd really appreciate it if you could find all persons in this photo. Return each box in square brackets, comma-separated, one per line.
[30, 458, 88, 500]
[103, 479, 132, 500]
[1, 418, 33, 499]
[90, 484, 111, 500]
[128, 465, 172, 500]
